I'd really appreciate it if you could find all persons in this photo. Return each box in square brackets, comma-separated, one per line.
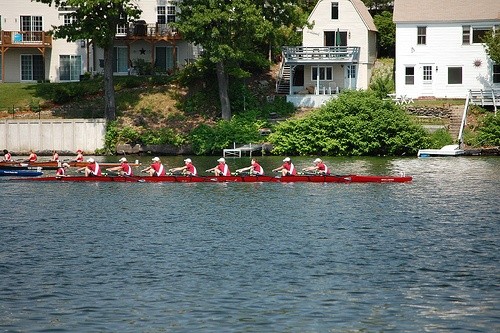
[209, 158, 230, 176]
[51, 150, 58, 161]
[56, 163, 70, 175]
[303, 158, 330, 176]
[75, 150, 83, 162]
[172, 158, 197, 177]
[78, 157, 101, 177]
[26, 150, 37, 161]
[2, 149, 11, 162]
[272, 157, 297, 176]
[239, 158, 264, 176]
[145, 157, 164, 176]
[109, 157, 133, 176]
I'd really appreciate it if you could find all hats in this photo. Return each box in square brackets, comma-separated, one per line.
[87, 158, 95, 163]
[119, 157, 128, 162]
[314, 158, 321, 162]
[283, 157, 290, 162]
[217, 158, 225, 162]
[184, 158, 191, 162]
[152, 157, 160, 161]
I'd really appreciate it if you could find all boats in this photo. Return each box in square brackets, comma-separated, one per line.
[0, 168, 44, 176]
[0, 160, 142, 171]
[0, 174, 412, 183]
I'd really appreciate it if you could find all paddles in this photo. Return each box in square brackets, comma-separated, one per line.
[194, 174, 217, 181]
[326, 174, 351, 181]
[108, 169, 147, 183]
